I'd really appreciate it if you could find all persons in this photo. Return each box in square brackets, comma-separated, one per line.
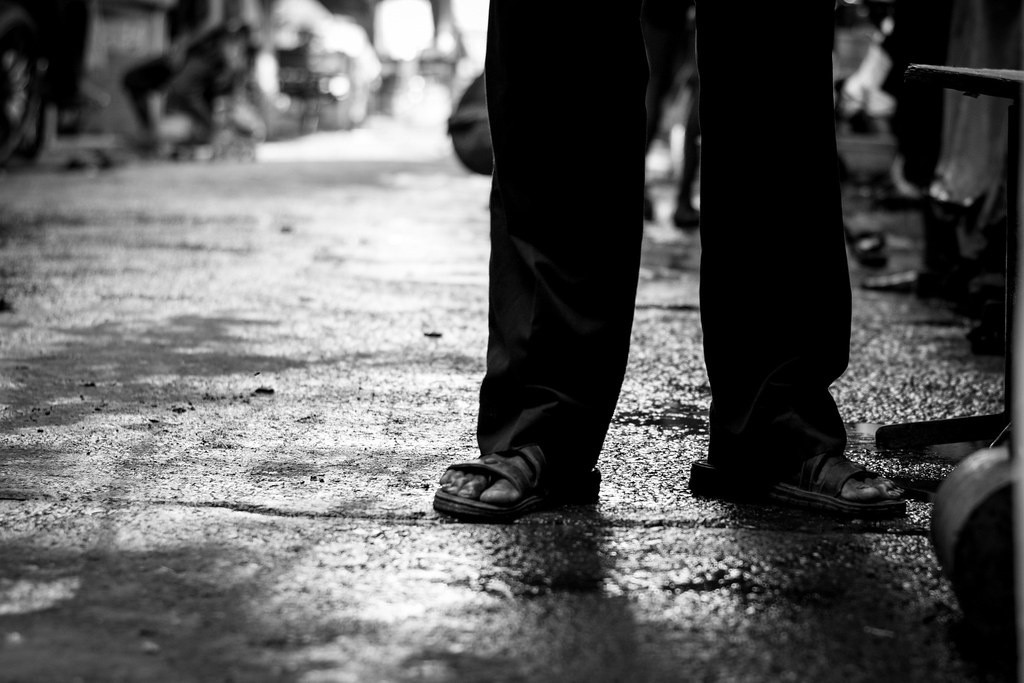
[432, 0, 906, 524]
[121, 0, 244, 163]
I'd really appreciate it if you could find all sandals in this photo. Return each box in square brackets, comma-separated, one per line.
[434, 443, 601, 523]
[689, 442, 907, 521]
